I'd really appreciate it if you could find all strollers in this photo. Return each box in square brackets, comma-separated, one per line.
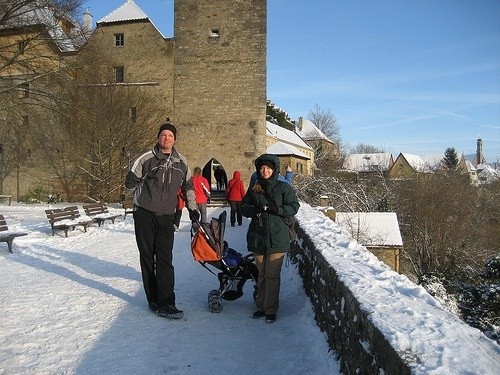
[191, 211, 259, 314]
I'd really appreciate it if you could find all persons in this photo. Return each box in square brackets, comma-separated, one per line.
[226, 171, 246, 227]
[222, 240, 256, 273]
[173, 187, 185, 232]
[214, 166, 227, 192]
[238, 154, 301, 323]
[249, 165, 293, 187]
[191, 167, 211, 227]
[124, 124, 200, 319]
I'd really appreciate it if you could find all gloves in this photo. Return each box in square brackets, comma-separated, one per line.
[207, 198, 210, 204]
[190, 209, 199, 221]
[267, 205, 278, 215]
[253, 207, 263, 218]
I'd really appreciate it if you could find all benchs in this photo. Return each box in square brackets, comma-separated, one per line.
[0, 214, 28, 254]
[123, 199, 134, 220]
[45, 206, 92, 237]
[82, 203, 121, 227]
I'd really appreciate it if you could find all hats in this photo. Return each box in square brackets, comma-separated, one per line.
[257, 161, 275, 170]
[157, 124, 177, 140]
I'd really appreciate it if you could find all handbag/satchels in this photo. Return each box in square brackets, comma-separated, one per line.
[287, 221, 297, 240]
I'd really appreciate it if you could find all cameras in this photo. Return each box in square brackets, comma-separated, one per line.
[261, 204, 269, 210]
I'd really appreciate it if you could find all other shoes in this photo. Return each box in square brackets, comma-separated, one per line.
[266, 313, 277, 322]
[250, 311, 264, 319]
[238, 223, 242, 227]
[159, 306, 184, 316]
[230, 223, 235, 228]
[173, 224, 179, 232]
[148, 302, 158, 310]
